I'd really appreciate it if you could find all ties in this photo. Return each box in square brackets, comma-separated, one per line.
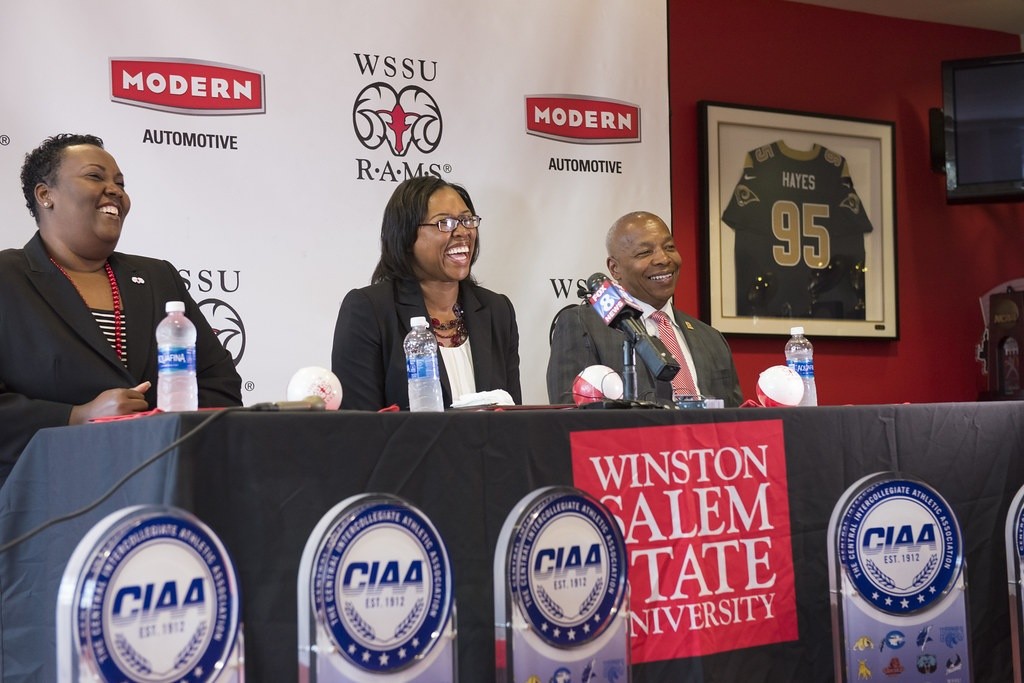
[649, 311, 698, 401]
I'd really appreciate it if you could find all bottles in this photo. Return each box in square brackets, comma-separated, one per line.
[156, 301, 199, 412]
[403, 316, 445, 411]
[784, 327, 818, 407]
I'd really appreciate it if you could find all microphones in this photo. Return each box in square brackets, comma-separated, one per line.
[587, 273, 681, 382]
[251, 396, 326, 412]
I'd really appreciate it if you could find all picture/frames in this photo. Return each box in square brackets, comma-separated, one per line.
[697, 99, 900, 342]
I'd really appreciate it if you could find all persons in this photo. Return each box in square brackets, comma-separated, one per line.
[0, 133, 242, 488]
[546, 211, 743, 407]
[331, 176, 522, 410]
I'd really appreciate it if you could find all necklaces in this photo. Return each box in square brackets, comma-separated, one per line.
[431, 313, 467, 348]
[52, 262, 121, 359]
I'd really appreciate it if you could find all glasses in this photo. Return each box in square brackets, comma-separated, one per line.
[418, 215, 482, 233]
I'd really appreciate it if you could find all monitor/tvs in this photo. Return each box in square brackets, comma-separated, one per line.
[941, 52, 1024, 206]
